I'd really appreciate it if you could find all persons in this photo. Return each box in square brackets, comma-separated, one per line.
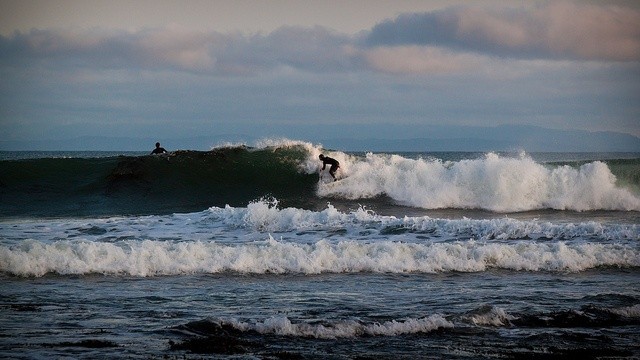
[150, 143, 168, 155]
[319, 154, 339, 181]
